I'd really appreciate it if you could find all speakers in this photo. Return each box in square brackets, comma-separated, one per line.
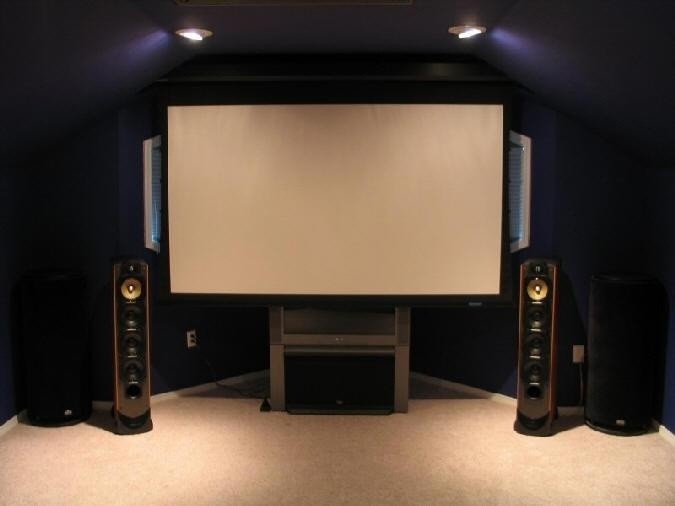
[583, 277, 667, 440]
[282, 345, 397, 416]
[516, 260, 557, 435]
[17, 274, 88, 427]
[111, 260, 153, 433]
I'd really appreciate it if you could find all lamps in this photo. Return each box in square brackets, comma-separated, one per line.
[447, 9, 488, 41]
[173, 14, 216, 43]
